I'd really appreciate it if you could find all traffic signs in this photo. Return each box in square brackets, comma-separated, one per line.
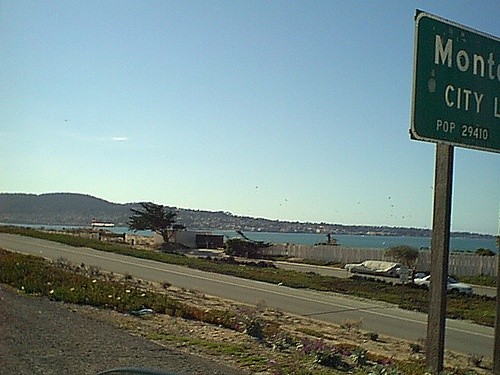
[410, 9, 500, 154]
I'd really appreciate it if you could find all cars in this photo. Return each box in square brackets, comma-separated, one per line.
[414, 274, 474, 294]
